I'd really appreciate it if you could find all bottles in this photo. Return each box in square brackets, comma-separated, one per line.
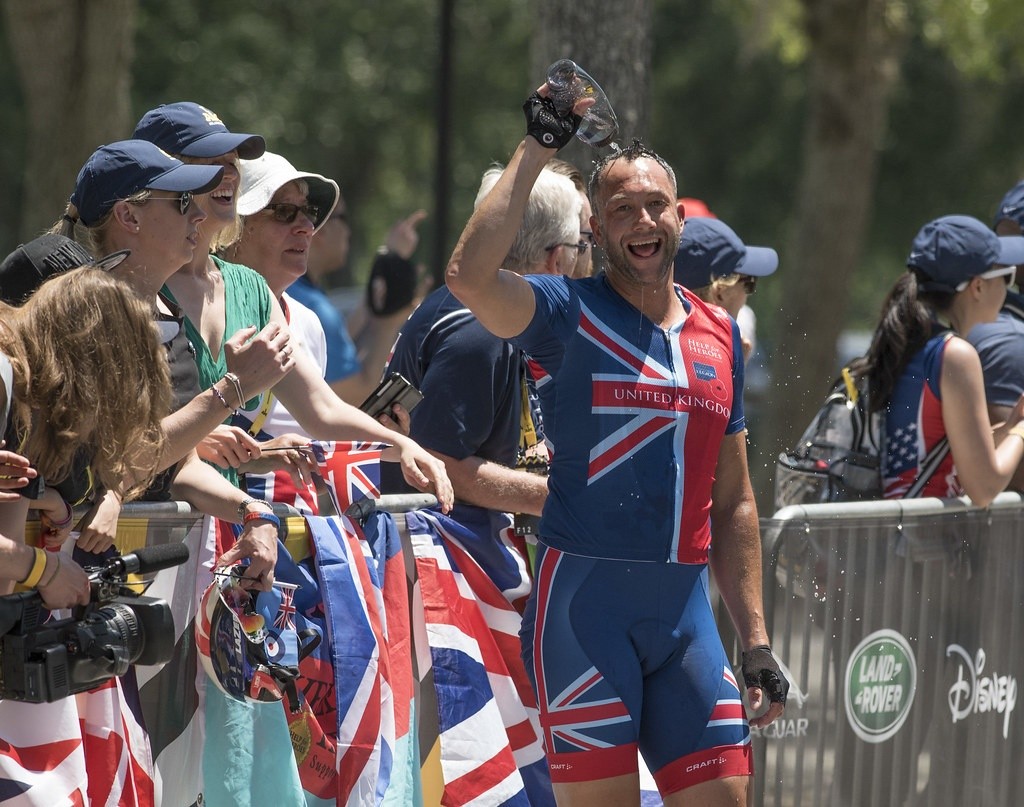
[546, 58, 619, 148]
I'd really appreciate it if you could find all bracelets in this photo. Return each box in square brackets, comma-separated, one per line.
[243, 512, 280, 530]
[224, 373, 246, 410]
[37, 501, 74, 548]
[17, 546, 46, 589]
[212, 383, 235, 414]
[237, 499, 274, 520]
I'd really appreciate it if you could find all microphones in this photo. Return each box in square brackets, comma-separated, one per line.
[108, 541, 189, 577]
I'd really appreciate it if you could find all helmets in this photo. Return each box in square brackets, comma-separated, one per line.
[196, 564, 285, 709]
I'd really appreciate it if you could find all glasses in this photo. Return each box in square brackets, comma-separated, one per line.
[580, 230, 598, 247]
[129, 190, 193, 216]
[736, 274, 757, 294]
[545, 238, 588, 255]
[956, 265, 1017, 292]
[265, 202, 323, 224]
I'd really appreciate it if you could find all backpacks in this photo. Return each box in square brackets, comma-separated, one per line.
[773, 329, 952, 514]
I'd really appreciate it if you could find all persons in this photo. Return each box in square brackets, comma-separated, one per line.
[131, 72, 788, 807]
[0, 440, 68, 553]
[0, 439, 91, 608]
[960, 178, 1024, 423]
[48, 140, 321, 485]
[2, 230, 279, 592]
[848, 215, 1024, 510]
[0, 264, 179, 506]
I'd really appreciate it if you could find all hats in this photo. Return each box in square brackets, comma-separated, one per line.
[70, 139, 225, 227]
[131, 102, 267, 160]
[906, 215, 1024, 292]
[1, 232, 133, 308]
[673, 217, 778, 290]
[236, 150, 340, 235]
[148, 321, 180, 344]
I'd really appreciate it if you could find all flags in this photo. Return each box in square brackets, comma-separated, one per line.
[301, 510, 417, 807]
[405, 506, 557, 807]
[272, 587, 296, 630]
[196, 459, 318, 807]
[308, 441, 395, 517]
